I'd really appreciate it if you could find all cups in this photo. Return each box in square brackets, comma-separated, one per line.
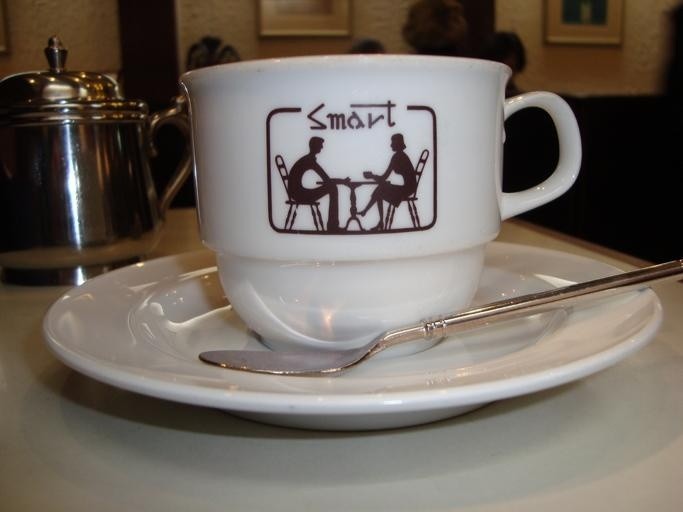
[177, 54, 582, 359]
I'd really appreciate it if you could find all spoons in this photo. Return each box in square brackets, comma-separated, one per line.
[198, 256, 683, 375]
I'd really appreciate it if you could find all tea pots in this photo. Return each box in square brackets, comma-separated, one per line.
[1, 38, 194, 286]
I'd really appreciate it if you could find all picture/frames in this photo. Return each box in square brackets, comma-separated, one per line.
[540, 2, 625, 51]
[255, 0, 354, 42]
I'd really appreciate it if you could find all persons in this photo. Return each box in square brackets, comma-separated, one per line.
[288, 137, 343, 232]
[357, 134, 417, 230]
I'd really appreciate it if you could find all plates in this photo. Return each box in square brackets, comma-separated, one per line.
[42, 241, 664, 431]
